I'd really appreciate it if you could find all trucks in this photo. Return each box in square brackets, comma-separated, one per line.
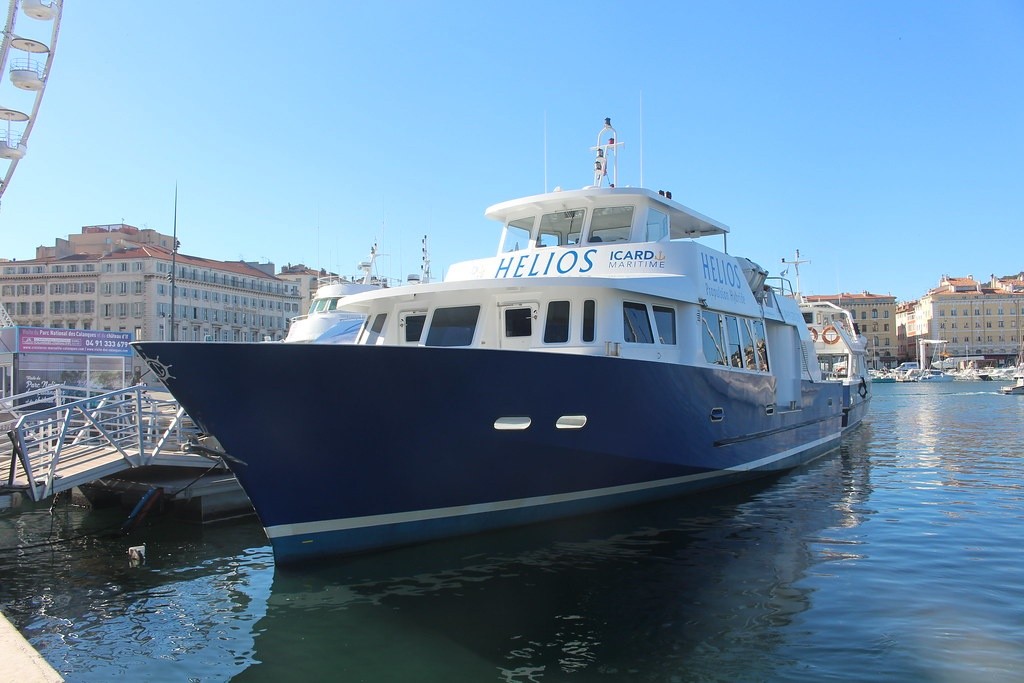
[943, 356, 985, 369]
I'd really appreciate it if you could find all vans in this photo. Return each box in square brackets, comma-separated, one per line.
[895, 362, 919, 372]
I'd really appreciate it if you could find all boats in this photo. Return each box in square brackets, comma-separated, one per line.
[779, 248, 873, 435]
[869, 363, 1024, 383]
[999, 378, 1024, 395]
[126, 115, 845, 567]
[279, 236, 435, 347]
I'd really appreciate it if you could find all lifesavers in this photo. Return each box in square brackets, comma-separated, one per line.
[822, 326, 841, 345]
[806, 327, 818, 344]
